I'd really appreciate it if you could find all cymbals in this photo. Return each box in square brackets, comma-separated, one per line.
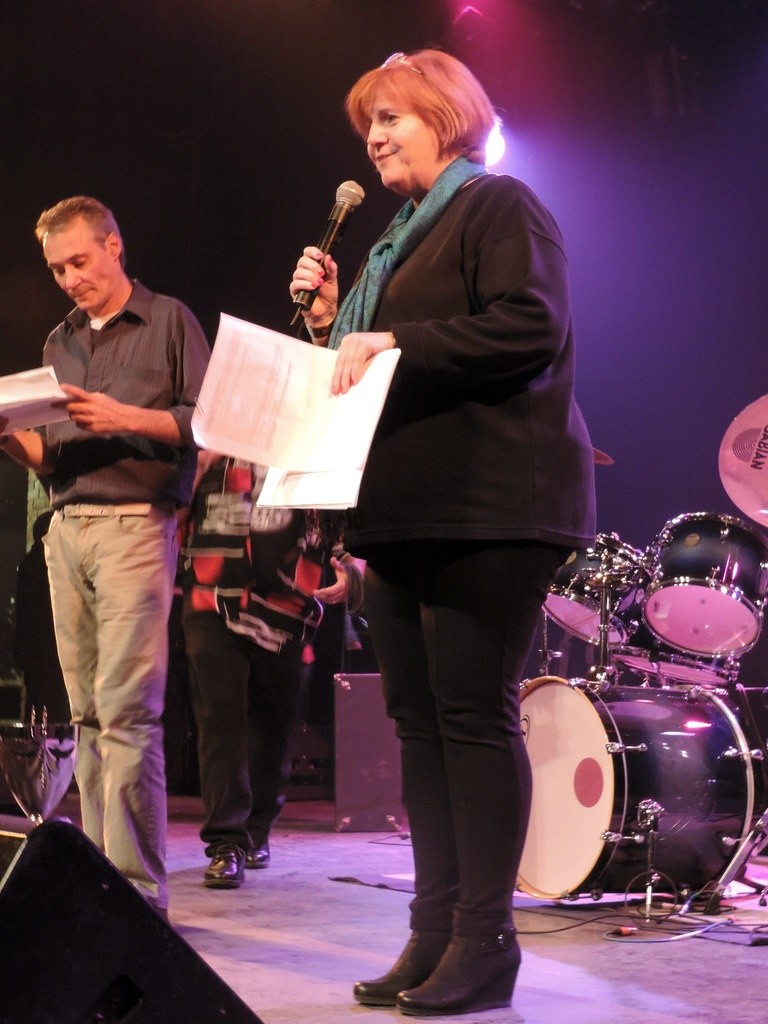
[716, 388, 768, 530]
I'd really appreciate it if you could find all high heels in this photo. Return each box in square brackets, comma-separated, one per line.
[396, 925, 522, 1015]
[354, 930, 453, 1005]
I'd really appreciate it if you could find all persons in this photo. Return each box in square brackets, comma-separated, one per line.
[0, 193, 210, 928]
[17, 363, 370, 891]
[285, 45, 610, 1014]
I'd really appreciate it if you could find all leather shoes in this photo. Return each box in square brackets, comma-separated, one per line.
[244, 840, 269, 868]
[203, 847, 245, 883]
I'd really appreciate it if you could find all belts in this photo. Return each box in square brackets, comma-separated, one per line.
[60, 502, 151, 516]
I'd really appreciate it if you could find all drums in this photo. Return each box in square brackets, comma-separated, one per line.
[640, 509, 768, 656]
[514, 671, 755, 905]
[543, 528, 650, 651]
[613, 639, 735, 690]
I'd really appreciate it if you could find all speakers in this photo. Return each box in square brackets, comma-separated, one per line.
[0, 814, 266, 1024]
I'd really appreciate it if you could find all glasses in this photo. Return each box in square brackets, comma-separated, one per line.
[382, 52, 426, 77]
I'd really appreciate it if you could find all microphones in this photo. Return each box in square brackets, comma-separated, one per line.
[289, 181, 365, 325]
